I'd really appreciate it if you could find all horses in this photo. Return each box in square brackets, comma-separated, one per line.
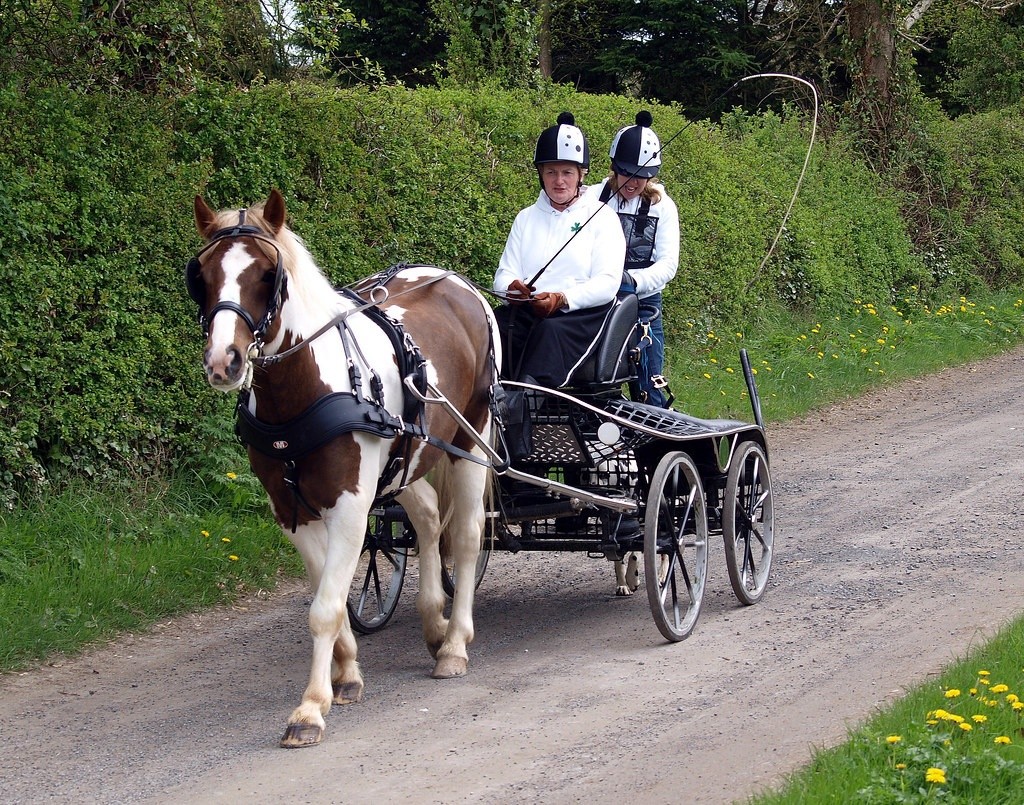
[184, 185, 503, 749]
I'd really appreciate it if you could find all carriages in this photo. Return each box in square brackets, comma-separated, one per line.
[185, 190, 776, 751]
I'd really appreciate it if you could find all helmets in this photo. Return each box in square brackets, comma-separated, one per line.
[609, 110, 662, 179]
[533, 112, 590, 169]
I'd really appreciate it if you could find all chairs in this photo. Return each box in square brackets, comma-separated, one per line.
[489, 292, 640, 386]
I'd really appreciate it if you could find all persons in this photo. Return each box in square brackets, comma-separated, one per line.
[592, 110, 681, 404]
[490, 113, 626, 412]
